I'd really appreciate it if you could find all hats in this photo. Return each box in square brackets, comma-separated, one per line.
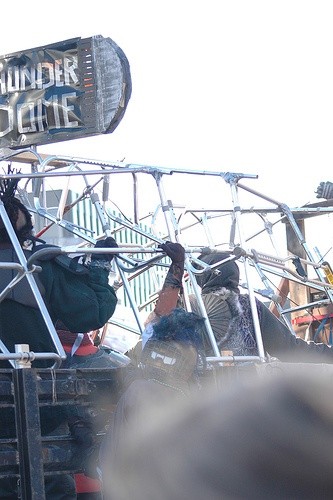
[192, 254, 240, 288]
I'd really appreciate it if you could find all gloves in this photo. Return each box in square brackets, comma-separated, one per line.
[91, 237, 118, 262]
[159, 241, 185, 288]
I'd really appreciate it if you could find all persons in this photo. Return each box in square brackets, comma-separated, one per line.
[0, 191, 118, 499]
[195, 251, 333, 364]
[50, 236, 186, 500]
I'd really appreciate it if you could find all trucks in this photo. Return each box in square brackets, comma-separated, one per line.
[0, 269, 333, 499]
